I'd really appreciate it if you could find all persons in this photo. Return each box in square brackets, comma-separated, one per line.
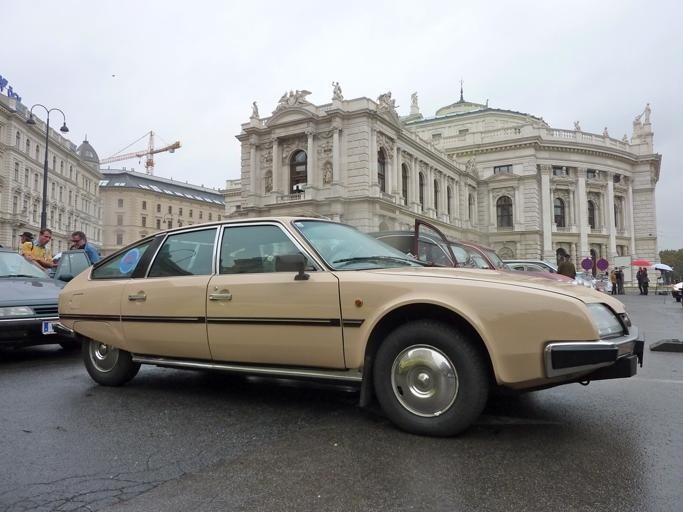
[331, 81, 342, 100]
[18, 228, 101, 275]
[641, 103, 651, 122]
[640, 267, 648, 295]
[610, 266, 625, 295]
[635, 267, 643, 295]
[557, 254, 576, 280]
[375, 91, 400, 127]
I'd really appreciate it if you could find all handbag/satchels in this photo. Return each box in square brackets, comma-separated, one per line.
[643, 278, 650, 283]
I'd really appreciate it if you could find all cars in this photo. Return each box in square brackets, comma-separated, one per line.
[672, 282, 683, 302]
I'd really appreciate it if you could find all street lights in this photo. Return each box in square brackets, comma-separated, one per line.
[26, 104, 68, 228]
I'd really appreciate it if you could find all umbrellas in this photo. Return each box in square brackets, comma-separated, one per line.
[652, 264, 672, 270]
[628, 260, 652, 267]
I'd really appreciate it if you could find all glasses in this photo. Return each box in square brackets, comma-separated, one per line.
[72, 238, 82, 243]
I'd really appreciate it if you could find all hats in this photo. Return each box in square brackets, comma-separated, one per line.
[18, 232, 33, 238]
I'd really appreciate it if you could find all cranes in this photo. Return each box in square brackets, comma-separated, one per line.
[99, 131, 182, 172]
